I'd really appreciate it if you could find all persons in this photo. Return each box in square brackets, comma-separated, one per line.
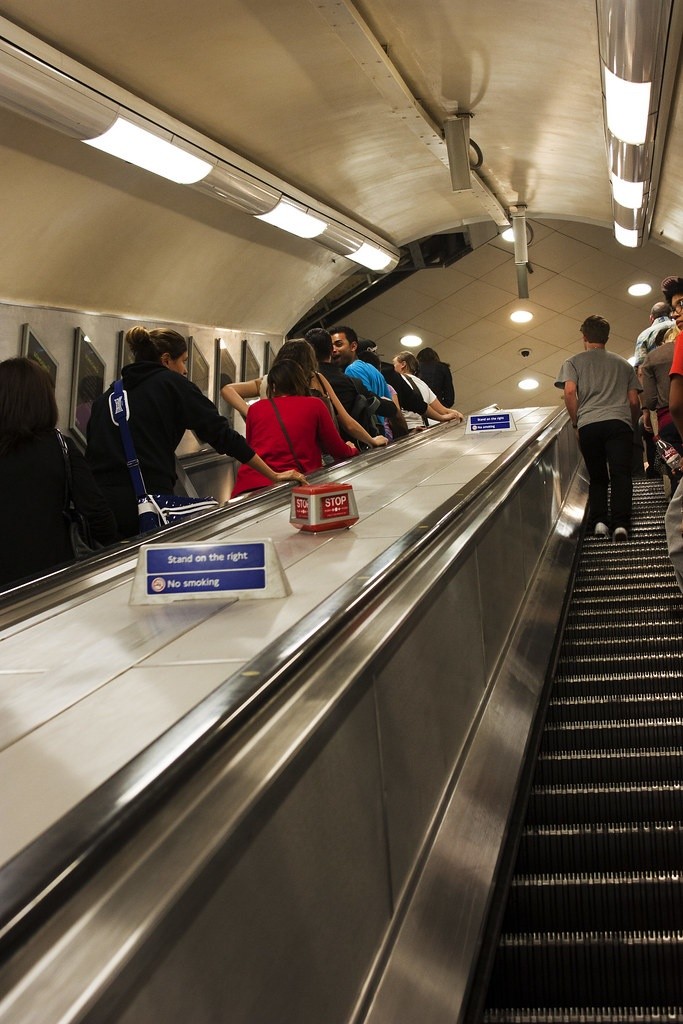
[553, 315, 644, 540]
[220, 324, 463, 522]
[633, 275, 683, 594]
[0, 356, 121, 584]
[83, 327, 311, 540]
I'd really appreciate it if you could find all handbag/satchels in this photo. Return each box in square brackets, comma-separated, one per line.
[66, 516, 99, 560]
[653, 439, 682, 478]
[136, 494, 218, 529]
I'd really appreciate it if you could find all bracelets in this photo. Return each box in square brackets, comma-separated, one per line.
[571, 424, 578, 429]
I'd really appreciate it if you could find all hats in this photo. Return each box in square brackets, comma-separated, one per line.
[661, 275, 683, 305]
[357, 338, 378, 352]
[579, 314, 610, 337]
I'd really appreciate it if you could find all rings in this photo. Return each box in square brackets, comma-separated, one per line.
[299, 475, 301, 477]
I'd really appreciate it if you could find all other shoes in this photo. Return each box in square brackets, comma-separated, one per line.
[643, 462, 651, 476]
[614, 527, 628, 541]
[594, 522, 609, 537]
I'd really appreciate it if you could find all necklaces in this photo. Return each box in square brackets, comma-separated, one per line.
[590, 346, 606, 349]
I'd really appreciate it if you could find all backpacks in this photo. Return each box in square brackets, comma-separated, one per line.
[348, 375, 385, 452]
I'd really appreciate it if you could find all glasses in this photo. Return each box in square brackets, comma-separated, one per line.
[668, 301, 683, 321]
